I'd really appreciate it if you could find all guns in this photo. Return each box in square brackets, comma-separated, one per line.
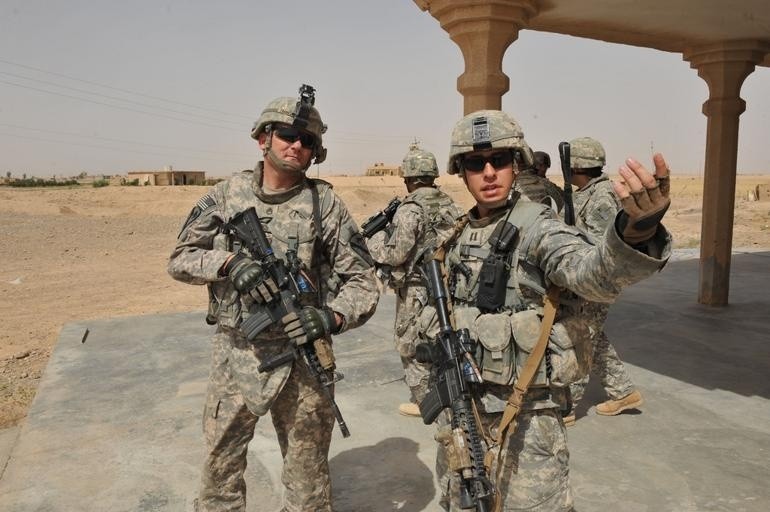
[406, 248, 497, 511]
[222, 207, 350, 439]
[559, 142, 575, 226]
[361, 196, 402, 238]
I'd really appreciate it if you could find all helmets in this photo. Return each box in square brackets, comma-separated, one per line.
[445, 109, 533, 176]
[569, 136, 607, 170]
[533, 150, 551, 169]
[249, 83, 328, 165]
[398, 150, 440, 179]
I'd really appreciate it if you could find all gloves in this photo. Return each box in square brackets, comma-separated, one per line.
[224, 251, 282, 311]
[277, 303, 338, 349]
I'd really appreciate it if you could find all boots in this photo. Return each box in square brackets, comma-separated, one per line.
[595, 389, 644, 416]
[398, 400, 423, 418]
[563, 412, 576, 428]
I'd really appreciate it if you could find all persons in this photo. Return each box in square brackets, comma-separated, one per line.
[166, 94, 384, 512]
[508, 168, 564, 213]
[365, 146, 460, 418]
[393, 107, 675, 512]
[532, 150, 551, 177]
[561, 136, 644, 427]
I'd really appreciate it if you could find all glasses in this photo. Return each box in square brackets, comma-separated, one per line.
[460, 150, 513, 173]
[271, 126, 315, 150]
